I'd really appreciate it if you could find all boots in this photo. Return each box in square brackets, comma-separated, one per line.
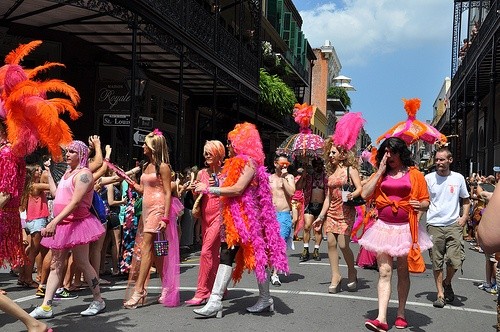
[247, 268, 275, 313]
[192, 263, 233, 319]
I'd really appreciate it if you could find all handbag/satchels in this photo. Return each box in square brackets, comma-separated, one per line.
[153, 229, 169, 255]
[340, 183, 365, 205]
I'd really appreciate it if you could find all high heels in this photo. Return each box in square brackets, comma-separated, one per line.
[327, 274, 343, 293]
[158, 287, 170, 304]
[124, 289, 148, 307]
[346, 268, 358, 289]
[184, 297, 207, 305]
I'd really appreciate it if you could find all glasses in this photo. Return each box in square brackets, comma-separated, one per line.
[279, 162, 290, 165]
[69, 150, 77, 153]
[226, 140, 231, 146]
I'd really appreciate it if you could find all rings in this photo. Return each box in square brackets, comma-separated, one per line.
[196, 186, 197, 187]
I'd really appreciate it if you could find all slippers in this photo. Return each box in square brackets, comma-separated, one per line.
[16, 278, 39, 288]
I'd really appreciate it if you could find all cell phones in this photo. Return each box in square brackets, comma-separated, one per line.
[41, 228, 56, 236]
[479, 183, 495, 192]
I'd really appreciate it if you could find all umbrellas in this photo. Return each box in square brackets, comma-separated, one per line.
[375, 98, 447, 148]
[276, 103, 325, 155]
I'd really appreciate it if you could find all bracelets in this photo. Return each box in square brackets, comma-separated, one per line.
[161, 217, 170, 224]
[384, 164, 386, 165]
[318, 215, 325, 219]
[131, 170, 135, 174]
[106, 158, 110, 160]
[204, 187, 208, 194]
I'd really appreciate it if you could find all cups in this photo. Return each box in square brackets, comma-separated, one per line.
[342, 191, 351, 202]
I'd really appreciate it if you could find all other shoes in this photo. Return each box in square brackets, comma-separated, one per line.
[299, 247, 310, 261]
[269, 269, 281, 287]
[64, 286, 79, 291]
[28, 306, 54, 318]
[434, 297, 444, 307]
[394, 315, 407, 329]
[74, 281, 88, 287]
[464, 233, 498, 294]
[54, 289, 78, 299]
[442, 279, 455, 301]
[314, 248, 321, 261]
[81, 300, 106, 316]
[36, 285, 45, 295]
[365, 319, 389, 332]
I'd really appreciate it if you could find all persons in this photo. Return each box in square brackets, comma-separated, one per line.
[193, 121, 288, 317]
[122, 129, 185, 309]
[0, 293, 53, 332]
[184, 140, 225, 305]
[418, 148, 470, 308]
[0, 115, 199, 300]
[269, 142, 369, 294]
[462, 167, 500, 332]
[361, 137, 429, 332]
[27, 140, 107, 319]
